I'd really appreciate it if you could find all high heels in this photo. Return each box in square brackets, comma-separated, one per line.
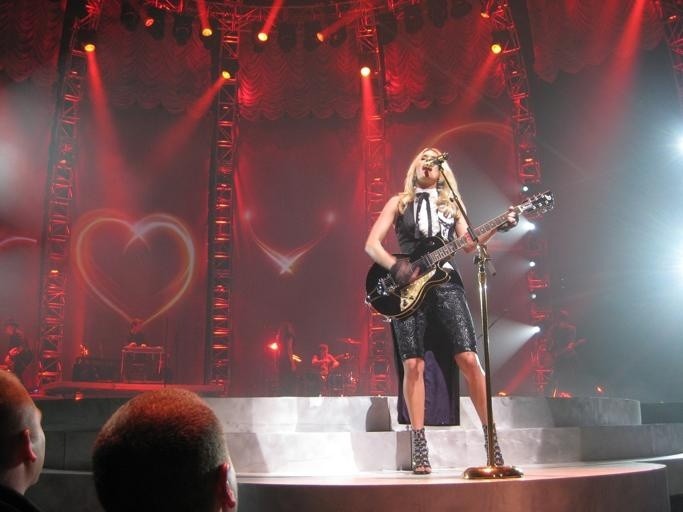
[410, 428, 431, 474]
[482, 423, 504, 467]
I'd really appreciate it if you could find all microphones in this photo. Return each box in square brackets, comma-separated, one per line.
[423, 152, 448, 169]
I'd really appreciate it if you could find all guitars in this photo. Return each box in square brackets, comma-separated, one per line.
[539, 338, 585, 367]
[365, 189, 555, 320]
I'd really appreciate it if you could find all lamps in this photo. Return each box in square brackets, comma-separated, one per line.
[82, 1, 510, 80]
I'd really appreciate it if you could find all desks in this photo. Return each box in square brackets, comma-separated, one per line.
[118, 344, 165, 385]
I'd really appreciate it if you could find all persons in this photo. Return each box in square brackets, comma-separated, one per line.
[361, 145, 505, 473]
[124, 318, 152, 367]
[269, 323, 298, 395]
[88, 386, 240, 512]
[0, 368, 46, 511]
[1, 315, 32, 389]
[307, 342, 338, 395]
[543, 308, 595, 396]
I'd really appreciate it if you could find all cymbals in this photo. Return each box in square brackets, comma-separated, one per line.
[338, 337, 364, 344]
